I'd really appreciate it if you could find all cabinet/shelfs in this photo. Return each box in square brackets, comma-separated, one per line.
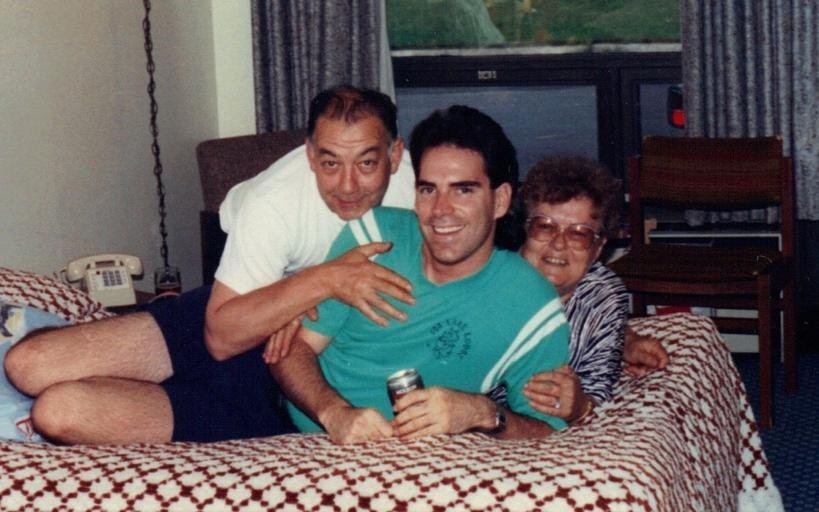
[607, 225, 786, 364]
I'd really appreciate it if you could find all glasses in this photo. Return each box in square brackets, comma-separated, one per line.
[525, 212, 596, 252]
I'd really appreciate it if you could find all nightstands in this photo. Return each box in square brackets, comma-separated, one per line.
[104, 283, 161, 315]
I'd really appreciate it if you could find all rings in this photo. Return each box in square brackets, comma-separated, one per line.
[551, 400, 562, 410]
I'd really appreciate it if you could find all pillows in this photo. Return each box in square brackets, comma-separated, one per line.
[1, 266, 101, 323]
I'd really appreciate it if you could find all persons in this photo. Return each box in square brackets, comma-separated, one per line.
[0, 104, 573, 446]
[260, 158, 632, 427]
[197, 80, 671, 379]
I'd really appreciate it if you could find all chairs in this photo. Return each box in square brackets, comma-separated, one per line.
[605, 137, 797, 428]
[196, 125, 309, 288]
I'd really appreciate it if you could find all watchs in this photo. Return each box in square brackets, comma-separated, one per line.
[489, 406, 507, 438]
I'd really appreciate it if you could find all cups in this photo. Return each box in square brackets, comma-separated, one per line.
[154, 266, 181, 297]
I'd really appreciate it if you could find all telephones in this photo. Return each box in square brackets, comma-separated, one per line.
[66, 254, 143, 308]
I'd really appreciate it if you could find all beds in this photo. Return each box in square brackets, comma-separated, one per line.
[1, 264, 781, 512]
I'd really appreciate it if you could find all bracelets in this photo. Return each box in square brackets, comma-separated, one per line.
[576, 398, 596, 422]
